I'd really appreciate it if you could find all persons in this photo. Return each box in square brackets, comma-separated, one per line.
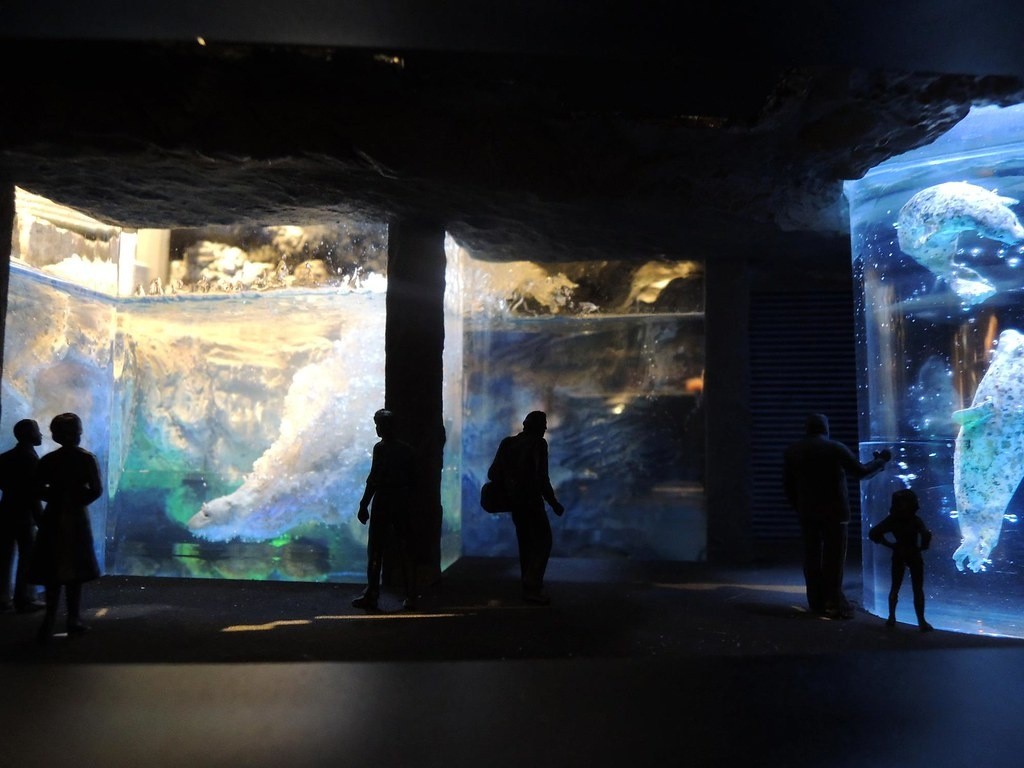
[0, 413, 103, 640]
[869, 489, 934, 632]
[782, 413, 891, 619]
[352, 409, 565, 610]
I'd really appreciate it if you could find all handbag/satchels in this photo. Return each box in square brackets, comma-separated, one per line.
[480, 483, 511, 513]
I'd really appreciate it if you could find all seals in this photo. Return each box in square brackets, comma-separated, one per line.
[188, 497, 236, 529]
[893, 181, 1024, 304]
[951, 328, 1023, 572]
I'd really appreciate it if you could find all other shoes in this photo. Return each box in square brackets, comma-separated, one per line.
[15, 599, 46, 610]
[920, 624, 932, 631]
[403, 598, 416, 611]
[67, 622, 92, 633]
[352, 592, 379, 610]
[1, 597, 15, 609]
[885, 618, 895, 625]
[820, 605, 853, 620]
[39, 624, 53, 638]
